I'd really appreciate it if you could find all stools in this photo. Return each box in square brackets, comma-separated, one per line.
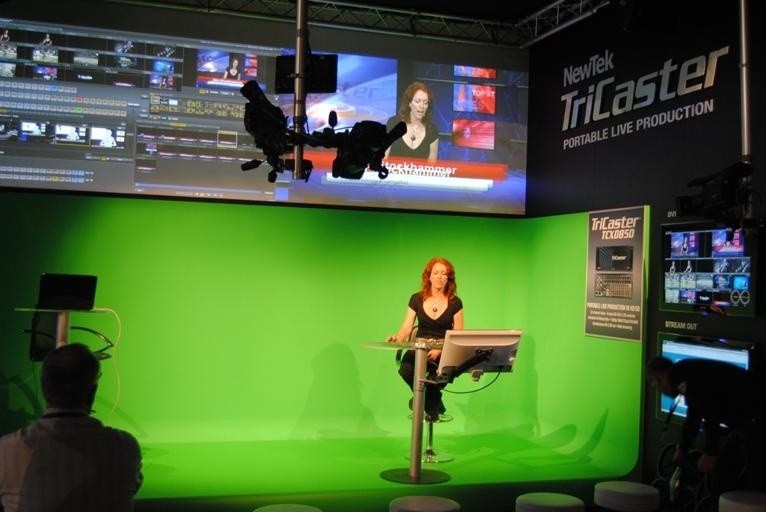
[516, 492, 584, 511]
[256, 504, 321, 512]
[390, 496, 460, 512]
[718, 492, 764, 512]
[593, 482, 658, 512]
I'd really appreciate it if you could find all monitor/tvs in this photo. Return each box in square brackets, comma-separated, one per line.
[659, 222, 758, 320]
[656, 329, 755, 435]
[436, 329, 520, 375]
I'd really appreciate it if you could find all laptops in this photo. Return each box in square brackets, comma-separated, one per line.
[33, 272, 97, 310]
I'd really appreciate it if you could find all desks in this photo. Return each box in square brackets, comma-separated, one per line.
[14, 307, 112, 348]
[367, 341, 451, 485]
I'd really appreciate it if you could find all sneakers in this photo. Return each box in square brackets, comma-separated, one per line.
[408, 391, 447, 422]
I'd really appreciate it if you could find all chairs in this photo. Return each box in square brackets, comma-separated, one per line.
[20, 312, 114, 363]
[396, 350, 456, 463]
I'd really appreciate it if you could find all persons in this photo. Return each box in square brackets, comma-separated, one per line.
[222, 58, 241, 81]
[386, 257, 465, 417]
[384, 81, 441, 164]
[0, 342, 143, 512]
[663, 228, 751, 304]
